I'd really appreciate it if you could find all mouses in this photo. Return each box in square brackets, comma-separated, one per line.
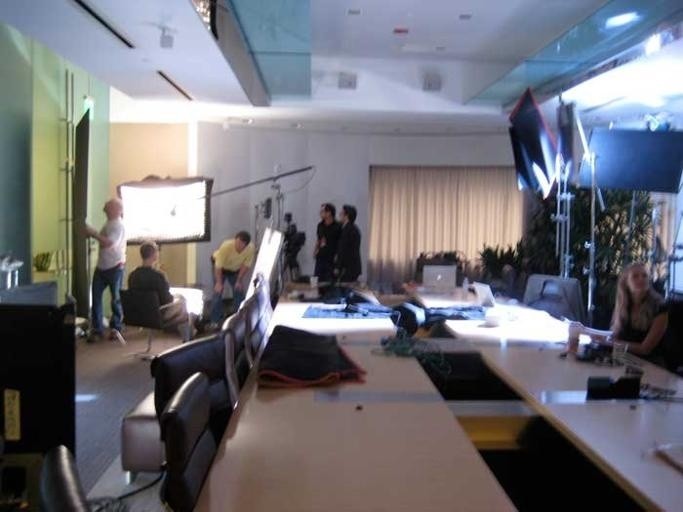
[506, 297, 519, 306]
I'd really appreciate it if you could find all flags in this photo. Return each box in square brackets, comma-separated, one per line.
[508, 86, 563, 201]
[557, 100, 590, 188]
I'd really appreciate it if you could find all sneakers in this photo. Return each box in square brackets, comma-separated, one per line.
[85, 329, 125, 343]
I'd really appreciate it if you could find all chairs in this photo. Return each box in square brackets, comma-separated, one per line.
[39, 445, 92, 511]
[119, 289, 189, 362]
[489, 266, 682, 377]
[150, 274, 273, 511]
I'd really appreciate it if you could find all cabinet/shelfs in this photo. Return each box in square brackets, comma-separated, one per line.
[0, 280, 75, 458]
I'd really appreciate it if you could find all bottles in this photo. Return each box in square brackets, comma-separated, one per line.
[567, 325, 581, 360]
[462, 277, 469, 300]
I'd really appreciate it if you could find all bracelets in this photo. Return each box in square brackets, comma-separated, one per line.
[624, 341, 629, 353]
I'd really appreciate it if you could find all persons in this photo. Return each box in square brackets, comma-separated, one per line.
[209, 232, 255, 330]
[127, 241, 173, 326]
[589, 263, 671, 365]
[86, 199, 128, 345]
[332, 204, 362, 293]
[313, 203, 342, 296]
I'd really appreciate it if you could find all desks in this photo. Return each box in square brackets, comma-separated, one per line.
[400, 282, 683, 512]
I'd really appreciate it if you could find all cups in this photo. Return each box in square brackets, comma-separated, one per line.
[612, 341, 628, 360]
[310, 276, 318, 287]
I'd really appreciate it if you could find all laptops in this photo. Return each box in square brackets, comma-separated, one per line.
[473, 280, 507, 308]
[416, 264, 457, 295]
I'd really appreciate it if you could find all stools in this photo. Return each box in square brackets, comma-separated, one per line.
[121, 390, 167, 485]
